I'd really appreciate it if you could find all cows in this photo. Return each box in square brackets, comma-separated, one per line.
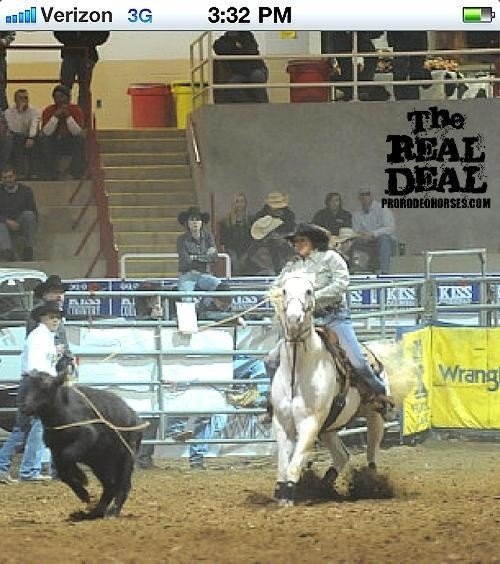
[18, 368, 144, 522]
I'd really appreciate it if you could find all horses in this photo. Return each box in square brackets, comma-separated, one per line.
[267, 262, 386, 509]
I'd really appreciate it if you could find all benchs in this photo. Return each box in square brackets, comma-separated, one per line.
[1, 153, 108, 278]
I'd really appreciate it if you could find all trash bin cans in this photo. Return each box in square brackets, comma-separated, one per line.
[127, 80, 208, 130]
[287, 59, 331, 103]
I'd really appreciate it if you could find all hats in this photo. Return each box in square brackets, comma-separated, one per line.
[250, 215, 285, 240]
[264, 192, 289, 209]
[284, 222, 330, 251]
[35, 274, 70, 299]
[31, 301, 66, 322]
[177, 207, 211, 225]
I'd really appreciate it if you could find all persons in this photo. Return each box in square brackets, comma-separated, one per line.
[25, 275, 75, 475]
[213, 31, 433, 103]
[0, 31, 109, 263]
[257, 222, 397, 424]
[177, 206, 221, 315]
[189, 283, 271, 469]
[0, 300, 66, 485]
[216, 187, 395, 277]
[134, 283, 164, 467]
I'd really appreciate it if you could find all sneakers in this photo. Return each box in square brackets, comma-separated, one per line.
[35, 473, 52, 481]
[0, 473, 19, 485]
